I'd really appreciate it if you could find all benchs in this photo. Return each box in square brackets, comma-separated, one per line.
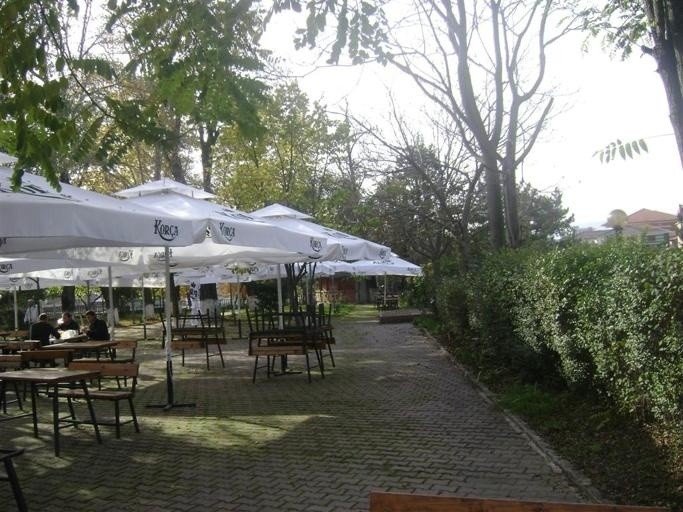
[364, 486, 675, 512]
[374, 293, 401, 311]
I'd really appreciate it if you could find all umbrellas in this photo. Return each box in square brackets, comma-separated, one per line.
[349, 251, 424, 306]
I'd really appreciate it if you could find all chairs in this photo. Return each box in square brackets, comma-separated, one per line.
[158, 304, 338, 386]
[0, 303, 142, 459]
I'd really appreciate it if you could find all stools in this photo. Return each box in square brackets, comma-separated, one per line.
[0, 447, 33, 512]
